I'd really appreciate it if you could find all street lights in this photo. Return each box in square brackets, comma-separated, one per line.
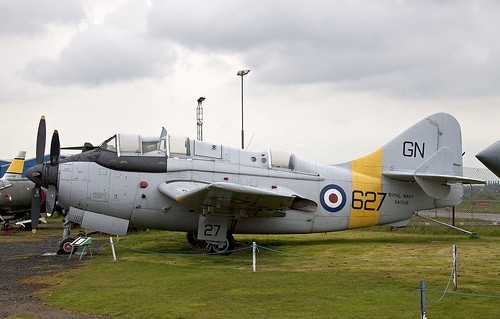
[236, 69, 251, 149]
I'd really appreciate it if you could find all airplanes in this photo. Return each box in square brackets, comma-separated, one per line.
[22, 112, 491, 256]
[0, 150, 60, 230]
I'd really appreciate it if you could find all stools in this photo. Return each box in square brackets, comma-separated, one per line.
[68, 245, 93, 261]
